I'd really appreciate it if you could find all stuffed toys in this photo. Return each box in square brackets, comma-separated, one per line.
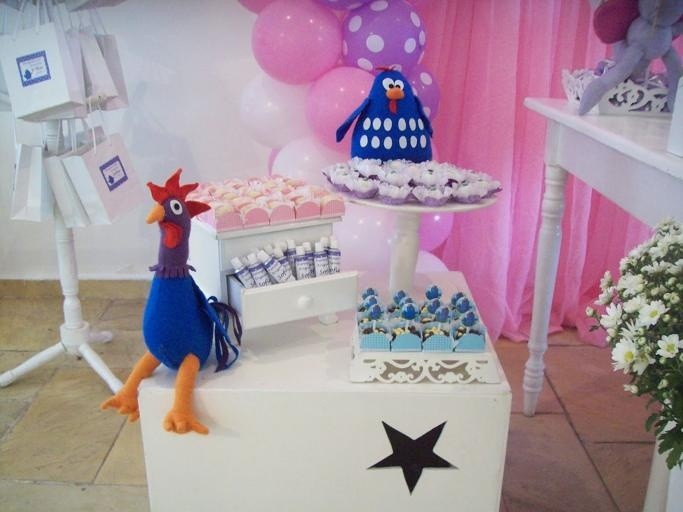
[101, 168, 243, 434]
[335, 66, 433, 164]
[576, 0, 683, 118]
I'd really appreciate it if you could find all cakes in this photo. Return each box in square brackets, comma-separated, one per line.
[183, 174, 347, 228]
[327, 150, 503, 205]
[357, 284, 487, 352]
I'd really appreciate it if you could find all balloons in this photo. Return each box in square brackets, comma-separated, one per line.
[318, 0, 373, 11]
[252, 0, 342, 85]
[407, 63, 439, 124]
[307, 66, 376, 154]
[270, 138, 454, 272]
[342, 0, 428, 78]
[239, 0, 273, 14]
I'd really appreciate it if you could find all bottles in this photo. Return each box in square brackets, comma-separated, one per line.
[230, 237, 340, 287]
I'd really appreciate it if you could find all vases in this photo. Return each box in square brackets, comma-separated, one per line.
[643, 400, 683, 512]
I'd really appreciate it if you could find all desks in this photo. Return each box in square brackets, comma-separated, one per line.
[523, 98, 682, 512]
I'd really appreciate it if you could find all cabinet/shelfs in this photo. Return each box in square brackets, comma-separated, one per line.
[137, 270, 511, 512]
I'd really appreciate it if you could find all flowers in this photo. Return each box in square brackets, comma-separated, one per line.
[584, 217, 682, 470]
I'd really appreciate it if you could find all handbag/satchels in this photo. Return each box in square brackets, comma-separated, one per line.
[1, 1, 127, 125]
[9, 116, 146, 231]
